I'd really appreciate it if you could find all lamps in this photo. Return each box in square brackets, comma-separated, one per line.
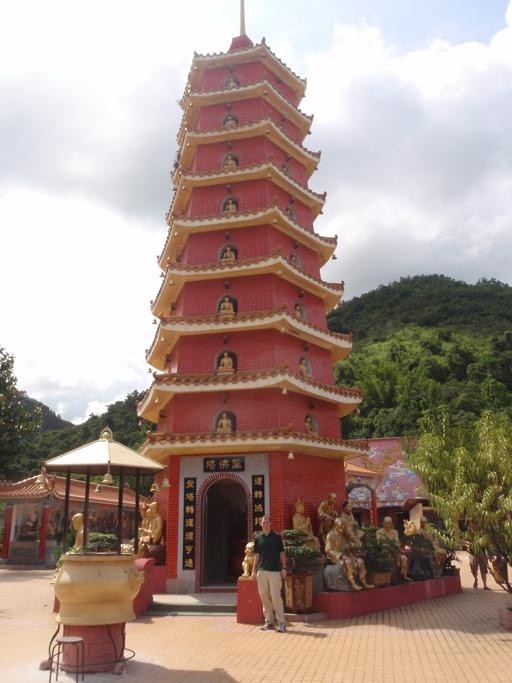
[94, 461, 115, 492]
[282, 387, 289, 394]
[288, 449, 294, 459]
[149, 479, 170, 492]
[35, 465, 50, 493]
[138, 419, 142, 426]
[154, 395, 160, 402]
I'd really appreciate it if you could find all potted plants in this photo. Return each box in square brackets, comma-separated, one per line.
[279, 529, 322, 613]
[400, 533, 435, 580]
[359, 526, 399, 587]
[441, 545, 460, 575]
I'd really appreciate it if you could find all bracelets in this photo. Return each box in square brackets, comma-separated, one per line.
[281, 568, 287, 569]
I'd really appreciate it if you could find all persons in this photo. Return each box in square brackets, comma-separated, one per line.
[130, 499, 165, 564]
[324, 516, 375, 591]
[216, 351, 234, 370]
[292, 495, 321, 552]
[225, 76, 238, 87]
[340, 500, 364, 540]
[299, 359, 308, 377]
[222, 245, 235, 260]
[219, 296, 235, 312]
[375, 515, 415, 580]
[295, 305, 301, 319]
[464, 521, 490, 589]
[305, 417, 317, 435]
[251, 514, 287, 633]
[223, 199, 238, 211]
[316, 492, 338, 545]
[419, 516, 447, 577]
[216, 412, 233, 433]
[224, 155, 237, 167]
[224, 113, 238, 127]
[51, 512, 90, 583]
[290, 255, 296, 265]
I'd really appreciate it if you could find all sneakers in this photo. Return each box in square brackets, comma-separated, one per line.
[277, 625, 286, 632]
[261, 623, 274, 630]
[473, 582, 490, 590]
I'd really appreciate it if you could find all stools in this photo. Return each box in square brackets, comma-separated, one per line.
[48, 636, 84, 683]
